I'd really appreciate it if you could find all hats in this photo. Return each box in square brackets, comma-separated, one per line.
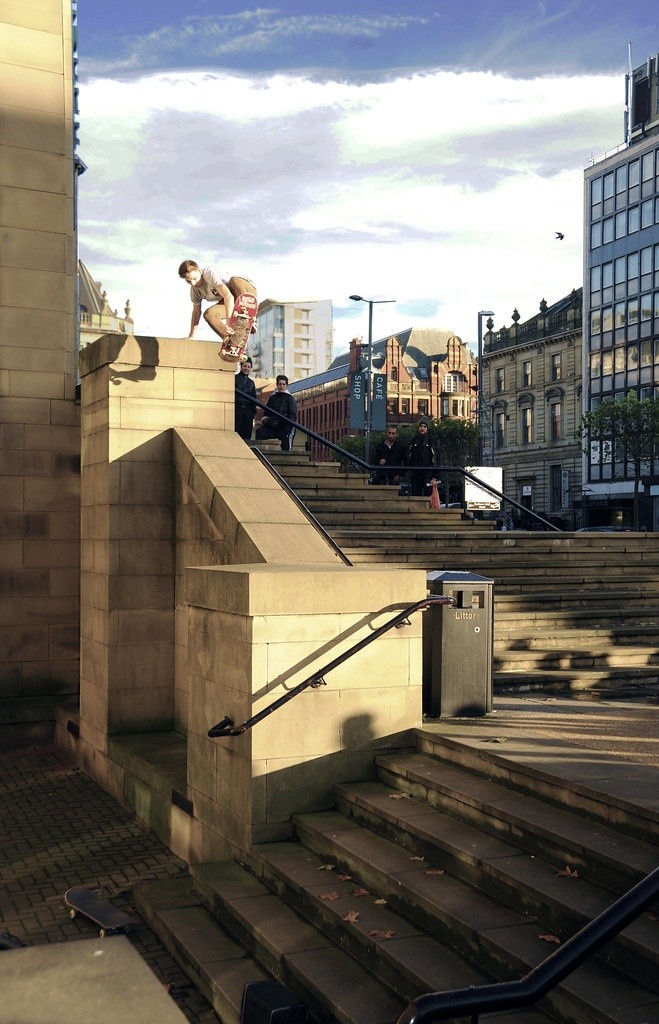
[418, 416, 429, 431]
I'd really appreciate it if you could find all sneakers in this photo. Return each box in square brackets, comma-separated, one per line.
[250, 320, 258, 334]
[239, 352, 248, 362]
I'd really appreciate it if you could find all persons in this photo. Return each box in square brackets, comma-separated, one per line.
[461, 506, 648, 534]
[371, 426, 407, 485]
[407, 421, 442, 497]
[234, 357, 257, 440]
[256, 375, 298, 452]
[178, 260, 258, 345]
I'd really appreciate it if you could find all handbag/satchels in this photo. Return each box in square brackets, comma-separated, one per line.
[430, 486, 440, 509]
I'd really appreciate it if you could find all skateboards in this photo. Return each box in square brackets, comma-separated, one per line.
[63, 886, 139, 938]
[218, 292, 258, 364]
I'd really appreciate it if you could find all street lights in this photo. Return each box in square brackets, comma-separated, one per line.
[350, 295, 396, 475]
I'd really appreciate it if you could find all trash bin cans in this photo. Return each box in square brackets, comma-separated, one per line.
[422, 570, 496, 718]
